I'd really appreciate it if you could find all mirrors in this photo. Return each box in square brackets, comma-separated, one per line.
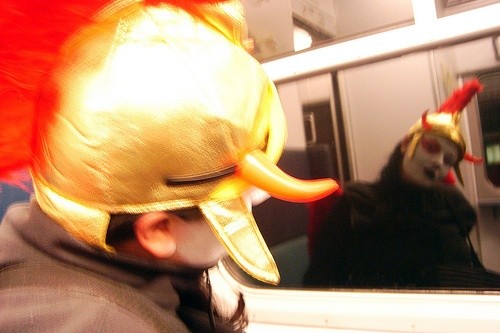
[210, 31, 500, 299]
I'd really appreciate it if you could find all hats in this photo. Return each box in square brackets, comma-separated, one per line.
[400, 111, 467, 191]
[28, 0, 287, 286]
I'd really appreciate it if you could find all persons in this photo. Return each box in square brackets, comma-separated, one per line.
[304, 112, 499, 289]
[1, 3, 286, 332]
